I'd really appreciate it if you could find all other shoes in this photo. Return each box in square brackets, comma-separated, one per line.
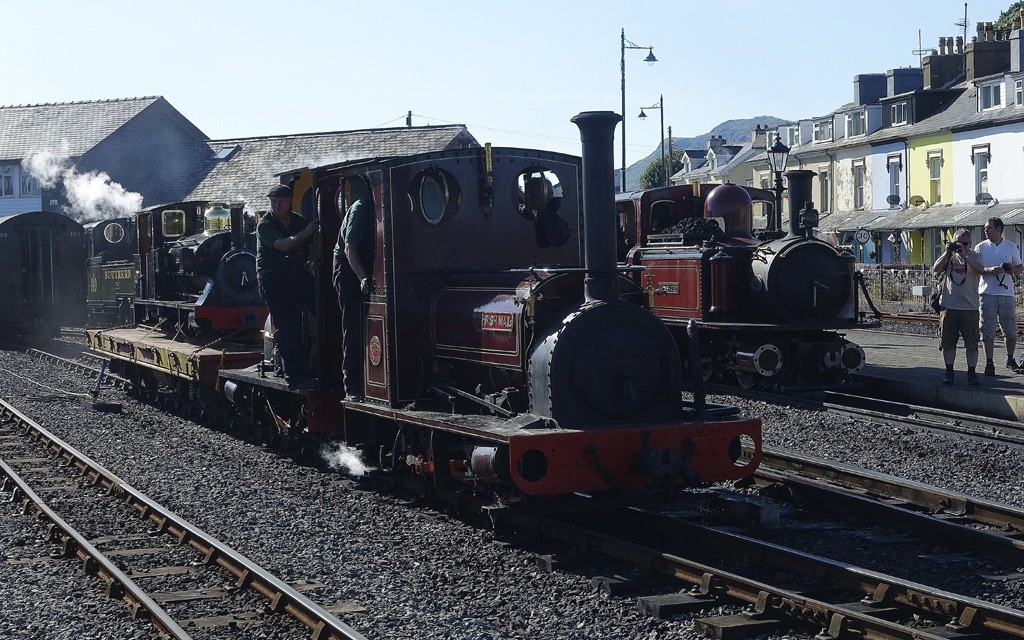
[1005, 358, 1019, 374]
[984, 365, 995, 376]
[967, 373, 981, 385]
[346, 392, 362, 402]
[1018, 361, 1024, 374]
[943, 370, 954, 384]
[290, 380, 318, 389]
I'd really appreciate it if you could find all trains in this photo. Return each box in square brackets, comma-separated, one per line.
[0, 211, 90, 337]
[614, 180, 866, 391]
[84, 109, 770, 537]
[83, 200, 256, 327]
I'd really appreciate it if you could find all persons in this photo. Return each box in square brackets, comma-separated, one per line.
[933, 229, 985, 385]
[331, 192, 377, 403]
[255, 184, 319, 389]
[974, 216, 1024, 374]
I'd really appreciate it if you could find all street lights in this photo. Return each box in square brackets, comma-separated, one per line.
[637, 92, 667, 186]
[766, 131, 791, 229]
[618, 27, 658, 192]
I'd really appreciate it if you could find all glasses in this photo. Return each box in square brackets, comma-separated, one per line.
[956, 241, 969, 247]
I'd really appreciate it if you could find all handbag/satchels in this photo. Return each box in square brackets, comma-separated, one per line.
[931, 288, 946, 316]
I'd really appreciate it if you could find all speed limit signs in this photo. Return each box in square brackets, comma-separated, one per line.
[856, 229, 871, 245]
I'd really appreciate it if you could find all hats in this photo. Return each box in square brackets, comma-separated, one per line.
[266, 185, 293, 198]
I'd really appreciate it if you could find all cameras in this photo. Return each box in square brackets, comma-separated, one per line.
[947, 243, 960, 251]
[1000, 261, 1011, 271]
